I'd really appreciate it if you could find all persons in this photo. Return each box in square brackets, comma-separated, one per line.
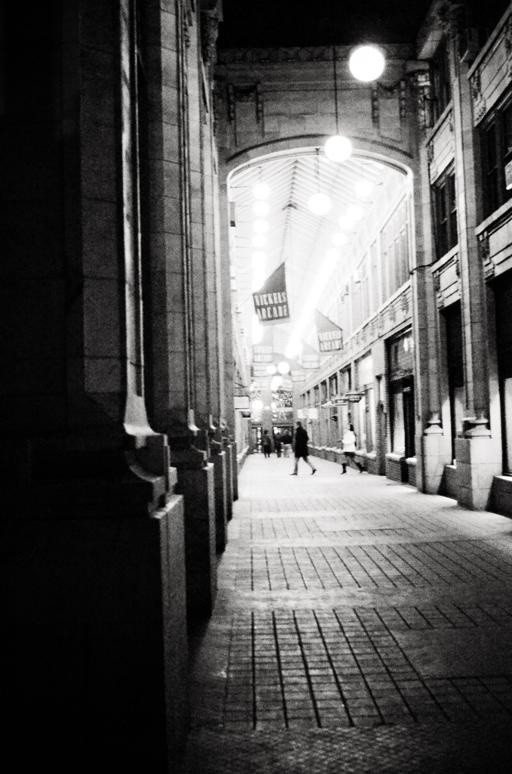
[260, 427, 292, 457]
[290, 420, 317, 474]
[335, 422, 365, 474]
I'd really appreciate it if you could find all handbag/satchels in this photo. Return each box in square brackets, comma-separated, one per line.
[336, 454, 347, 464]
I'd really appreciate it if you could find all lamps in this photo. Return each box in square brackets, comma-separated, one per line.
[324, 41, 353, 163]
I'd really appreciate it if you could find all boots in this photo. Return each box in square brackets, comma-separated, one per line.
[340, 462, 365, 474]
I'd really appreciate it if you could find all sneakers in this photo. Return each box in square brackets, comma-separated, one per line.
[290, 468, 317, 475]
